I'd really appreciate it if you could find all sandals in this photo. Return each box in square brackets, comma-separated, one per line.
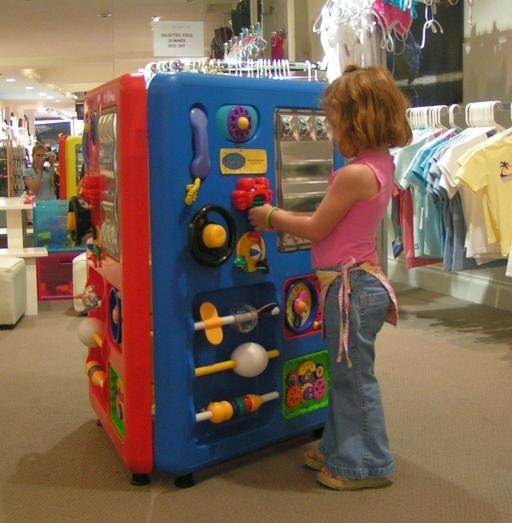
[303, 447, 393, 491]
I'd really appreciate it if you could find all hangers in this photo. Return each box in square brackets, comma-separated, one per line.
[223, 22, 268, 61]
[312, 0, 371, 34]
[404, 100, 511, 144]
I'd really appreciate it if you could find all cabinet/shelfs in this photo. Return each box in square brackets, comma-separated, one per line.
[0, 139, 15, 198]
[0, 195, 49, 317]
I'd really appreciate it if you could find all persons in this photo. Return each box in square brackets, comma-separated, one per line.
[22, 145, 55, 201]
[247, 64, 414, 489]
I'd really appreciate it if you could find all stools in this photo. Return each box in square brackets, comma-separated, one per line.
[0, 256, 27, 327]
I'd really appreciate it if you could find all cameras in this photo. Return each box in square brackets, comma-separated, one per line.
[44, 156, 50, 161]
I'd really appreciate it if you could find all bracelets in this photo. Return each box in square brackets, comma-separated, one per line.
[264, 207, 278, 228]
